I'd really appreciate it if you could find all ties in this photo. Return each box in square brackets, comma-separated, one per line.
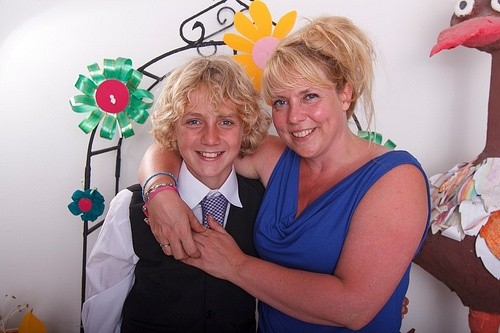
[200, 194, 229, 231]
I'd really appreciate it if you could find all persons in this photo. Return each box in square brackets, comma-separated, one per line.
[137, 16, 433, 333]
[81, 56, 267, 333]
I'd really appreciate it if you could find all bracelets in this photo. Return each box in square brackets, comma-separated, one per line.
[142, 172, 180, 220]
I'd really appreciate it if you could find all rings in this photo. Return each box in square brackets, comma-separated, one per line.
[161, 244, 170, 247]
